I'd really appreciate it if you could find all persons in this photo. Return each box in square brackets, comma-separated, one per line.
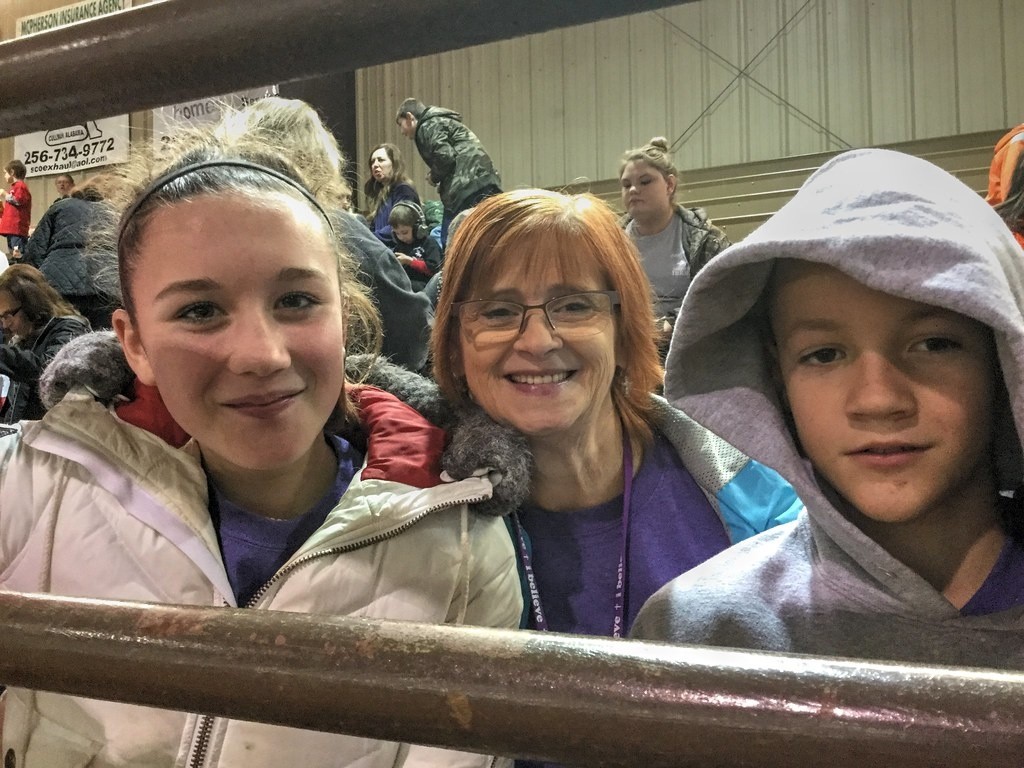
[627, 149, 1024, 672]
[430, 189, 806, 767]
[0, 143, 524, 768]
[0, 98, 1024, 436]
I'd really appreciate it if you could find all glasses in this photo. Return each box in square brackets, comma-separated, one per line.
[452, 290, 620, 340]
[0, 306, 22, 323]
